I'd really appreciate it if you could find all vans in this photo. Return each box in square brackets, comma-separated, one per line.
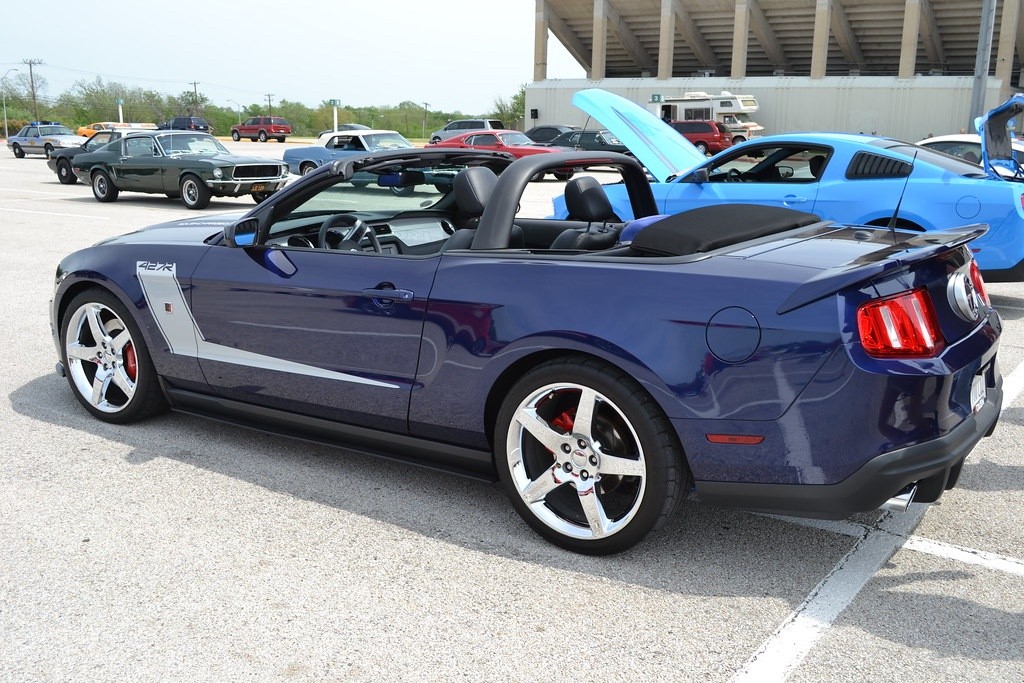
[428, 118, 505, 145]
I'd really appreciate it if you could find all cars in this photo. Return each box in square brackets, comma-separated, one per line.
[71, 130, 289, 210]
[792, 132, 1024, 180]
[547, 129, 645, 172]
[47, 128, 154, 185]
[539, 86, 1024, 285]
[7, 121, 98, 158]
[77, 122, 118, 138]
[524, 125, 582, 143]
[423, 130, 577, 182]
[318, 123, 372, 139]
[281, 130, 452, 199]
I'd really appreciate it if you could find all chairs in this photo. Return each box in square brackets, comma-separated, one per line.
[443, 167, 524, 251]
[549, 176, 620, 255]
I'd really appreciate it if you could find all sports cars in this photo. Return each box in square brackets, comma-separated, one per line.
[48, 148, 1004, 557]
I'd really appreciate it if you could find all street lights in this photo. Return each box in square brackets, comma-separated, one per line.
[329, 99, 341, 146]
[2, 69, 18, 138]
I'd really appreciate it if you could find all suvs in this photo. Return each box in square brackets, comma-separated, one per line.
[158, 117, 210, 135]
[667, 119, 733, 155]
[231, 116, 292, 142]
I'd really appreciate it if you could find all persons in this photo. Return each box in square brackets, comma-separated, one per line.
[808, 155, 825, 176]
[960, 128, 966, 134]
[965, 151, 978, 162]
[928, 133, 933, 138]
[662, 115, 671, 123]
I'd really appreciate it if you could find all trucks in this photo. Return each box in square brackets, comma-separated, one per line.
[648, 91, 765, 146]
